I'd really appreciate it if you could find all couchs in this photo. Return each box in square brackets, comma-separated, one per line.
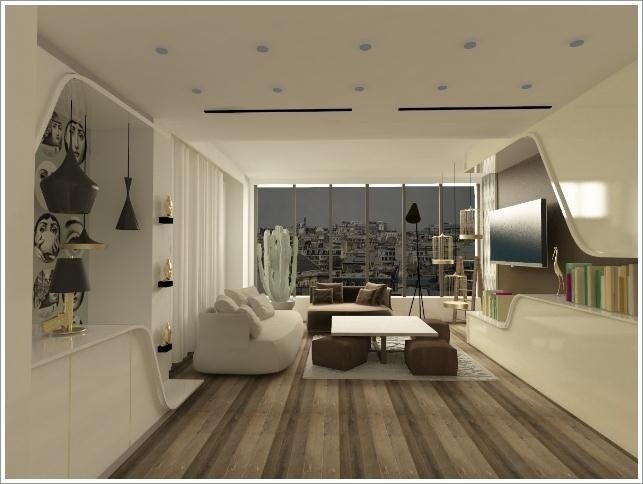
[193, 287, 304, 375]
[306, 285, 392, 335]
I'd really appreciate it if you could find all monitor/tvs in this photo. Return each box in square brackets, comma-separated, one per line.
[489, 198, 549, 269]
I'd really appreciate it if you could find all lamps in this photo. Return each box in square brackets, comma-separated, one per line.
[460, 172, 482, 239]
[434, 184, 454, 264]
[49, 257, 91, 335]
[115, 122, 140, 230]
[443, 162, 468, 309]
[40, 100, 100, 214]
[406, 203, 426, 319]
[60, 115, 107, 249]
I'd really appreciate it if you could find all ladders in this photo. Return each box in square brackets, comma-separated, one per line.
[452, 256, 478, 324]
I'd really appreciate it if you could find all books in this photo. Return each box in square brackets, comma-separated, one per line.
[479, 289, 517, 323]
[563, 263, 638, 321]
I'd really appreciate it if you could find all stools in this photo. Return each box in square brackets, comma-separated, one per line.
[312, 336, 371, 371]
[411, 319, 450, 344]
[405, 338, 458, 376]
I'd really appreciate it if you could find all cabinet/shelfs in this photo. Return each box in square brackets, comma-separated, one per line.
[158, 217, 173, 352]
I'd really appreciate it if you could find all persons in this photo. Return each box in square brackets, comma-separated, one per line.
[34, 213, 61, 264]
[62, 290, 86, 325]
[42, 110, 67, 158]
[36, 161, 58, 179]
[34, 270, 60, 317]
[64, 218, 84, 258]
[63, 119, 89, 164]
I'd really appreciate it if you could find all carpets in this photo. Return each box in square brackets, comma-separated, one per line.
[302, 335, 499, 382]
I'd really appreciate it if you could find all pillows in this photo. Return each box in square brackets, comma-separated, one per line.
[316, 283, 343, 303]
[356, 288, 376, 306]
[312, 288, 333, 305]
[216, 287, 275, 337]
[364, 282, 387, 305]
